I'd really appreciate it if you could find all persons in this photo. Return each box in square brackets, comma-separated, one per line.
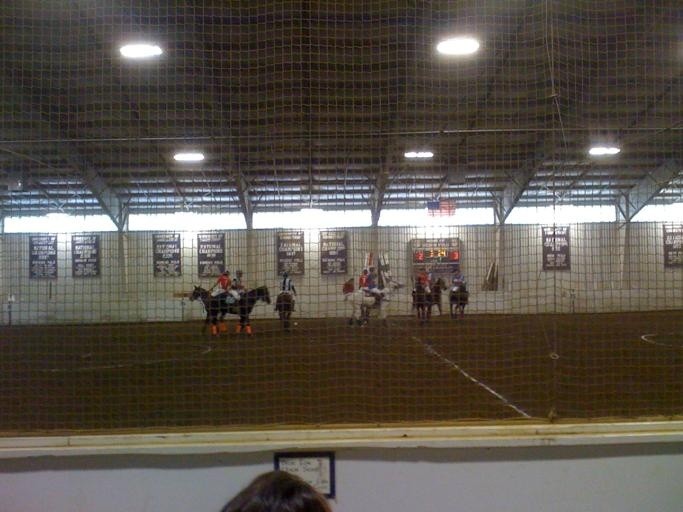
[274, 272, 298, 311]
[221, 471, 333, 512]
[451, 269, 466, 292]
[209, 270, 245, 306]
[359, 267, 384, 302]
[414, 268, 432, 294]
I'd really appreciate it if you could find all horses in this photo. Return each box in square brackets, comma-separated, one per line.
[277, 293, 293, 334]
[449, 281, 469, 319]
[344, 279, 406, 329]
[412, 277, 447, 321]
[189, 284, 271, 340]
